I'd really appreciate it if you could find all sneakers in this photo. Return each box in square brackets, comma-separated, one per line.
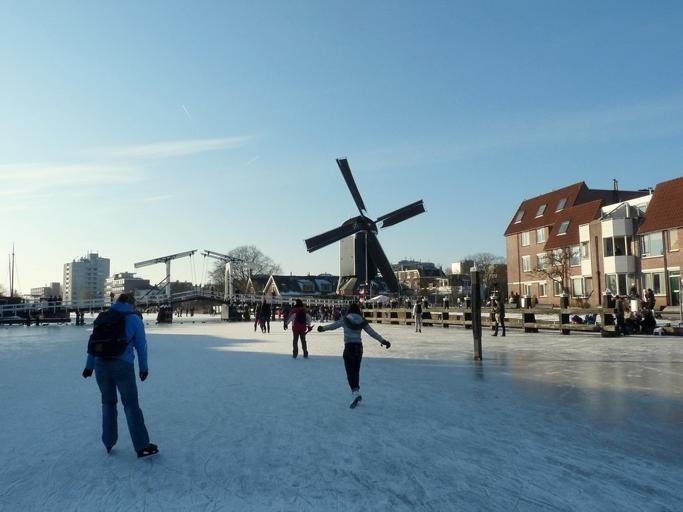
[349, 390, 362, 408]
[136, 443, 157, 455]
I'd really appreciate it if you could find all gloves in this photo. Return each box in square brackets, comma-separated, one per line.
[82, 368, 92, 378]
[139, 371, 147, 381]
[382, 340, 390, 348]
[283, 324, 287, 330]
[317, 326, 323, 332]
[307, 326, 312, 331]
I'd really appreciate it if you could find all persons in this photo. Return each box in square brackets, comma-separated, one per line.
[412, 300, 423, 333]
[47, 294, 62, 306]
[147, 306, 195, 317]
[231, 299, 398, 334]
[490, 299, 505, 336]
[283, 299, 312, 358]
[571, 288, 656, 336]
[82, 293, 157, 454]
[317, 303, 391, 409]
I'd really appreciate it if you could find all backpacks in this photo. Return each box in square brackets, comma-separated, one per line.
[415, 304, 422, 314]
[86, 307, 143, 358]
[295, 308, 306, 323]
